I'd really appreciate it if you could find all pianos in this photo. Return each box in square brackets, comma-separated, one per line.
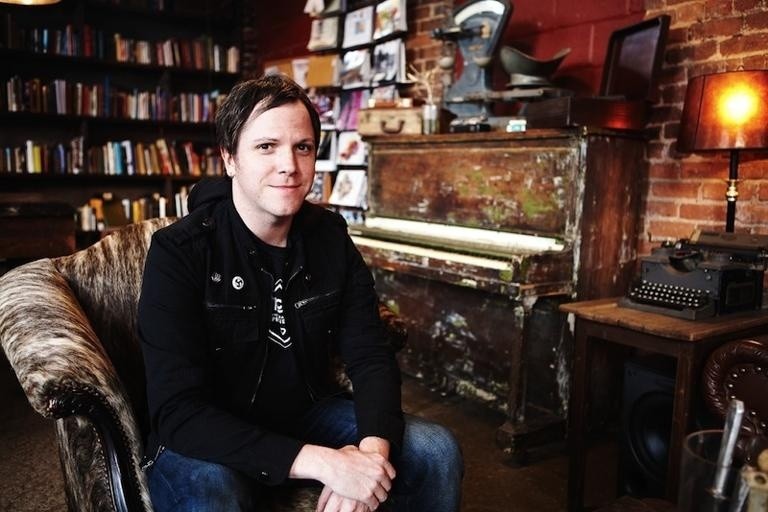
[348, 127, 649, 465]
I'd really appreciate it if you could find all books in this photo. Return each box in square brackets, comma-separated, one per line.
[28, 21, 238, 74]
[6, 76, 228, 124]
[261, 0, 409, 241]
[75, 186, 190, 233]
[5, 136, 223, 176]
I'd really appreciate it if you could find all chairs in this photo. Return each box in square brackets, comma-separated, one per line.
[1, 215, 408, 512]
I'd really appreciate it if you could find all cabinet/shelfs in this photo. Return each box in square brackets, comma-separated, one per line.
[292, 0, 418, 226]
[2, 1, 244, 246]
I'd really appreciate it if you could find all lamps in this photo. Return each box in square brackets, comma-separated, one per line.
[676, 68, 766, 234]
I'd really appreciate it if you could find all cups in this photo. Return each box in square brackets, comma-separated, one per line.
[677, 429, 761, 512]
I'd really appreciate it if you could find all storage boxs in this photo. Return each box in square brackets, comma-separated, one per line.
[356, 105, 423, 138]
[525, 95, 652, 130]
[2, 202, 77, 263]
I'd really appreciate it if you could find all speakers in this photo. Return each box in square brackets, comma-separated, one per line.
[618, 356, 726, 502]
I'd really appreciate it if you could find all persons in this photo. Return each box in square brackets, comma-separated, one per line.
[140, 73, 466, 512]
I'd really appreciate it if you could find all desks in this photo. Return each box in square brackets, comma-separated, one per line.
[557, 294, 768, 510]
